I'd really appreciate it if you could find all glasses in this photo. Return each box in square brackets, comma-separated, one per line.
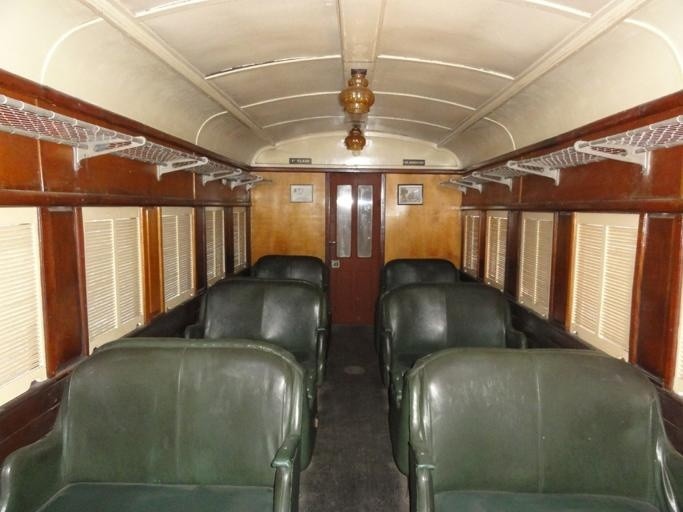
[340, 68, 376, 126]
[341, 124, 366, 158]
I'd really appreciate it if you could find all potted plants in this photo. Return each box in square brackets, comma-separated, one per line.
[395, 182, 424, 206]
[288, 182, 314, 205]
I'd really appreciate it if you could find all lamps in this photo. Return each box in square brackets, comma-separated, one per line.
[373, 257, 461, 386]
[250, 254, 334, 383]
[373, 283, 538, 478]
[180, 279, 322, 471]
[0, 336, 311, 512]
[399, 345, 682, 512]
